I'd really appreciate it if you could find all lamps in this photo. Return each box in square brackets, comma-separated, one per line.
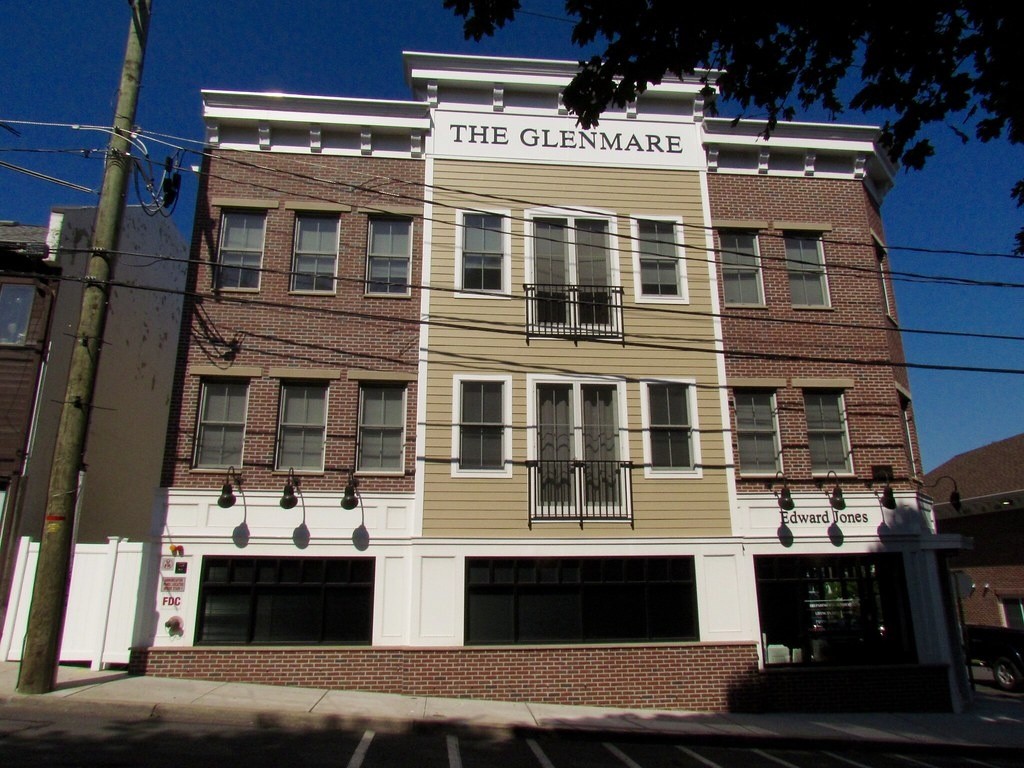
[341, 469, 360, 510]
[765, 471, 794, 510]
[919, 476, 961, 513]
[279, 467, 301, 509]
[816, 471, 846, 510]
[218, 466, 244, 508]
[865, 470, 897, 509]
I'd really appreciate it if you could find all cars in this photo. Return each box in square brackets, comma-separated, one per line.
[963, 620, 1024, 694]
